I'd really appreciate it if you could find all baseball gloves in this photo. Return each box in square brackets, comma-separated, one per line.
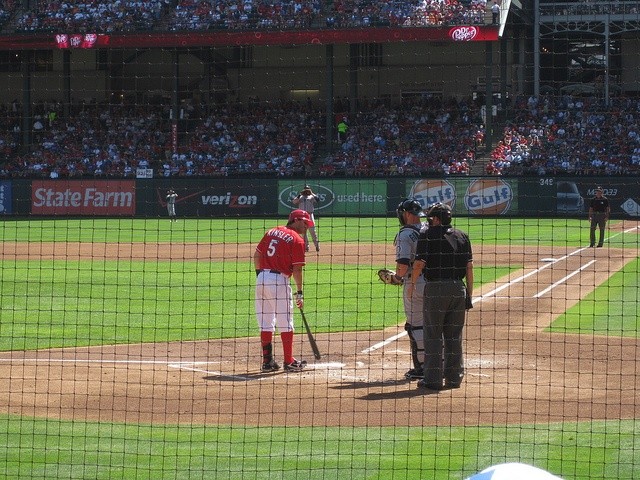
[378, 268, 404, 287]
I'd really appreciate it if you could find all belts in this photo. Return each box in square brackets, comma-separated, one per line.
[260, 270, 280, 274]
[408, 274, 421, 278]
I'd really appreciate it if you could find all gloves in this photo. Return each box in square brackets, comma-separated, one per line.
[296, 291, 303, 309]
[466, 295, 473, 309]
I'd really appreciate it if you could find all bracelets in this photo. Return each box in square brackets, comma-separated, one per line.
[297, 195, 301, 198]
[293, 290, 303, 295]
[395, 274, 402, 280]
[256, 269, 264, 277]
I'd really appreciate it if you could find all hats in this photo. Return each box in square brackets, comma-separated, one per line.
[597, 186, 603, 192]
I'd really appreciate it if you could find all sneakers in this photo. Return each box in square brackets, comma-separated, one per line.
[283, 357, 307, 372]
[597, 241, 602, 247]
[445, 379, 460, 388]
[405, 369, 425, 379]
[590, 241, 594, 247]
[261, 360, 279, 372]
[417, 378, 443, 390]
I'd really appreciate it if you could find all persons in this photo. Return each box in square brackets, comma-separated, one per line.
[409, 203, 473, 390]
[378, 201, 429, 378]
[16, 1, 171, 34]
[486, 93, 640, 177]
[254, 209, 313, 372]
[293, 185, 321, 254]
[174, 0, 322, 29]
[160, 98, 322, 176]
[588, 187, 610, 247]
[490, 2, 500, 24]
[0, 1, 20, 34]
[325, 0, 487, 27]
[166, 190, 178, 222]
[1, 101, 160, 177]
[320, 93, 487, 175]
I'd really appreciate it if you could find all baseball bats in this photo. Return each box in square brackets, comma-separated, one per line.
[292, 292, 322, 360]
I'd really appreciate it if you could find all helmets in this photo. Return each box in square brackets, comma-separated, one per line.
[289, 210, 314, 228]
[396, 199, 422, 226]
[425, 202, 452, 229]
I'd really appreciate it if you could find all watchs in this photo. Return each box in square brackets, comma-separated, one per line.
[410, 281, 415, 286]
